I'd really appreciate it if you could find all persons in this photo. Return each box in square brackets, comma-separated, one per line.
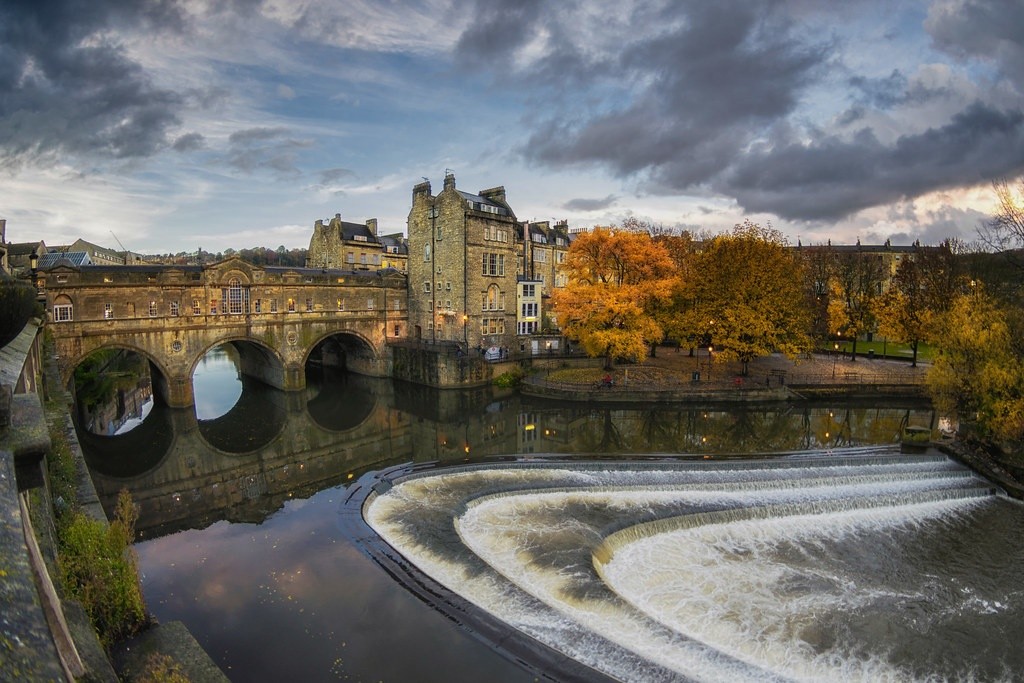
[842, 347, 847, 359]
[598, 372, 612, 389]
[735, 376, 748, 400]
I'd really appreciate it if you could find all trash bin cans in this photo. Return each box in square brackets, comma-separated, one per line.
[692, 371, 701, 380]
[869, 350, 874, 360]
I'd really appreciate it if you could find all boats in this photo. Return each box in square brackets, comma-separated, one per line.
[901, 425, 931, 454]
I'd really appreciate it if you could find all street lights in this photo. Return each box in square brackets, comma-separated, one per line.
[825, 408, 833, 438]
[463, 316, 468, 341]
[702, 412, 707, 442]
[464, 422, 470, 454]
[707, 346, 713, 380]
[831, 328, 841, 379]
[547, 341, 550, 376]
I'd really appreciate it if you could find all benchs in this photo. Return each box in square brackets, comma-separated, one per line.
[591, 381, 615, 391]
[767, 369, 787, 385]
[844, 373, 858, 380]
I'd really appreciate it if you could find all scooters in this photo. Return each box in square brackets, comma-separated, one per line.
[591, 379, 619, 391]
